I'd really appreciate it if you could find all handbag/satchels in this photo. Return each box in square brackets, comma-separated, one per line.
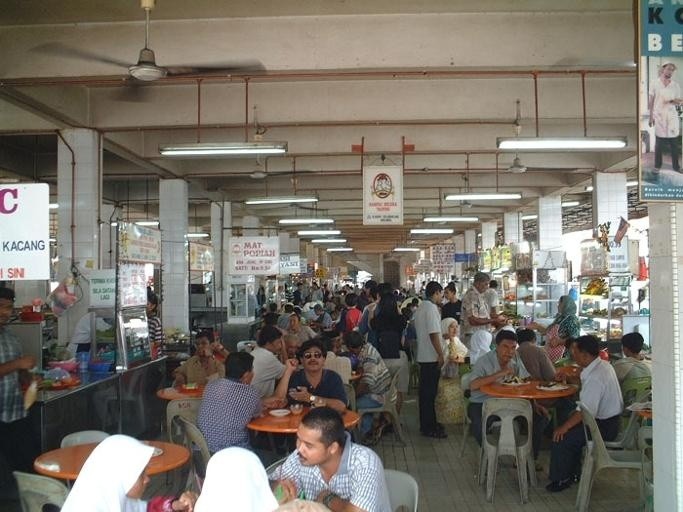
[434, 353, 464, 425]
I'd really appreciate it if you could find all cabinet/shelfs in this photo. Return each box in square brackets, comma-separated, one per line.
[577, 271, 634, 354]
[514, 248, 569, 324]
[88, 306, 151, 372]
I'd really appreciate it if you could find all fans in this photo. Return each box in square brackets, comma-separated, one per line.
[32, 0, 264, 96]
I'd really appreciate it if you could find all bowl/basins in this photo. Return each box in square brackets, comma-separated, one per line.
[18, 311, 43, 322]
[48, 361, 79, 373]
[88, 363, 113, 372]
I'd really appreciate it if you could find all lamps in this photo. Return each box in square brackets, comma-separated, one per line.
[160, 80, 355, 252]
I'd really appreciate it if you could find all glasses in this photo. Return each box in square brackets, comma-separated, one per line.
[302, 351, 322, 359]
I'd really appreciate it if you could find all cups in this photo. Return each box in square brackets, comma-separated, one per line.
[290, 404, 303, 415]
[77, 352, 89, 373]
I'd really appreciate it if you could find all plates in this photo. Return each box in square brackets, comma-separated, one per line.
[19, 375, 79, 392]
[268, 409, 291, 416]
[535, 384, 569, 391]
[150, 446, 162, 458]
[500, 380, 531, 386]
[173, 384, 201, 394]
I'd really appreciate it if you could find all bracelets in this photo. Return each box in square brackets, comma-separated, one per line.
[324, 491, 337, 508]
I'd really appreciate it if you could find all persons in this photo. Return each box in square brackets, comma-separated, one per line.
[160, 270, 652, 471]
[1, 286, 39, 511]
[543, 335, 626, 493]
[194, 444, 280, 511]
[146, 288, 165, 350]
[266, 404, 393, 511]
[645, 47, 683, 176]
[55, 433, 200, 511]
[60, 306, 116, 358]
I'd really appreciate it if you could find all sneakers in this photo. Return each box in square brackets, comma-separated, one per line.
[513, 456, 542, 471]
[420, 423, 448, 438]
[361, 420, 386, 446]
[546, 473, 579, 493]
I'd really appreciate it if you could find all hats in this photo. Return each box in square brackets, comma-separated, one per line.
[309, 304, 323, 310]
